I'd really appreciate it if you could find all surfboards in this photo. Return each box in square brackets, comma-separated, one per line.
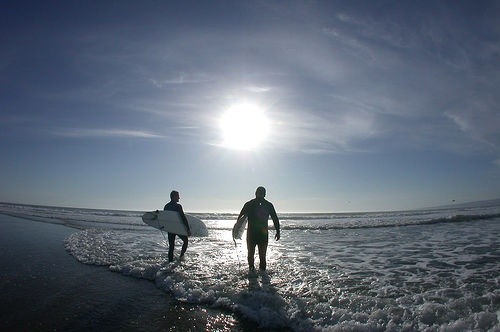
[232, 214, 249, 239]
[141, 210, 209, 238]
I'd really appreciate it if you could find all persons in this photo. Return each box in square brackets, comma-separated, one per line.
[164, 190, 191, 262]
[237, 186, 280, 270]
